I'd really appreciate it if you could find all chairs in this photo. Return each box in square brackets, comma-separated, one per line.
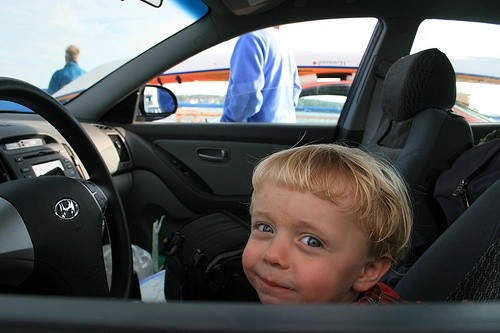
[393, 180, 500, 304]
[362, 48, 473, 236]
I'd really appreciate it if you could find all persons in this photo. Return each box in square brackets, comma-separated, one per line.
[241, 142, 414, 306]
[48, 46, 88, 95]
[220, 24, 302, 124]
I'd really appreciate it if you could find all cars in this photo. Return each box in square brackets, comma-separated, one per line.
[300, 82, 494, 126]
